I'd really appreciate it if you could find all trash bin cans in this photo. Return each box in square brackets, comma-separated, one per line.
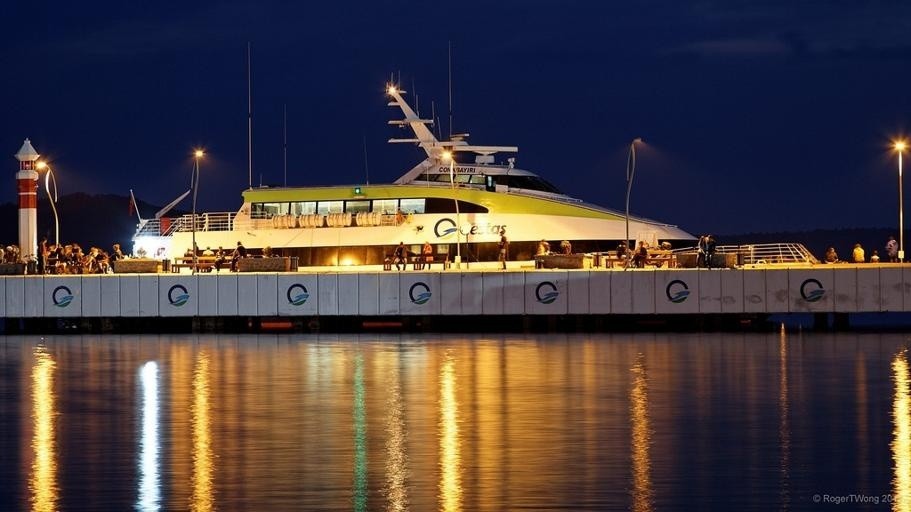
[736, 254, 744, 269]
[28, 261, 36, 275]
[163, 257, 170, 273]
[591, 252, 604, 270]
[291, 256, 299, 272]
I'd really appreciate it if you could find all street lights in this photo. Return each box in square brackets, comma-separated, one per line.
[37, 161, 61, 249]
[441, 148, 463, 266]
[890, 137, 910, 262]
[191, 147, 204, 274]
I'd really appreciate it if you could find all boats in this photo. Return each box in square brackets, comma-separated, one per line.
[118, 70, 819, 264]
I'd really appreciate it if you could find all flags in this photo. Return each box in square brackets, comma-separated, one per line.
[128, 193, 135, 217]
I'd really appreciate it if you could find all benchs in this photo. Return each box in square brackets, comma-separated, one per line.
[675, 253, 735, 268]
[171, 256, 251, 273]
[237, 257, 291, 271]
[112, 260, 158, 272]
[45, 258, 72, 271]
[384, 253, 448, 270]
[605, 250, 674, 268]
[534, 254, 583, 268]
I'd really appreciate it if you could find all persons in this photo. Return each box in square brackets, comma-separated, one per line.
[629, 241, 647, 268]
[497, 231, 508, 269]
[183, 241, 247, 273]
[617, 241, 627, 259]
[824, 247, 839, 264]
[394, 242, 409, 272]
[870, 250, 880, 262]
[851, 242, 865, 263]
[0, 237, 122, 274]
[885, 236, 898, 262]
[421, 242, 432, 269]
[695, 235, 716, 268]
